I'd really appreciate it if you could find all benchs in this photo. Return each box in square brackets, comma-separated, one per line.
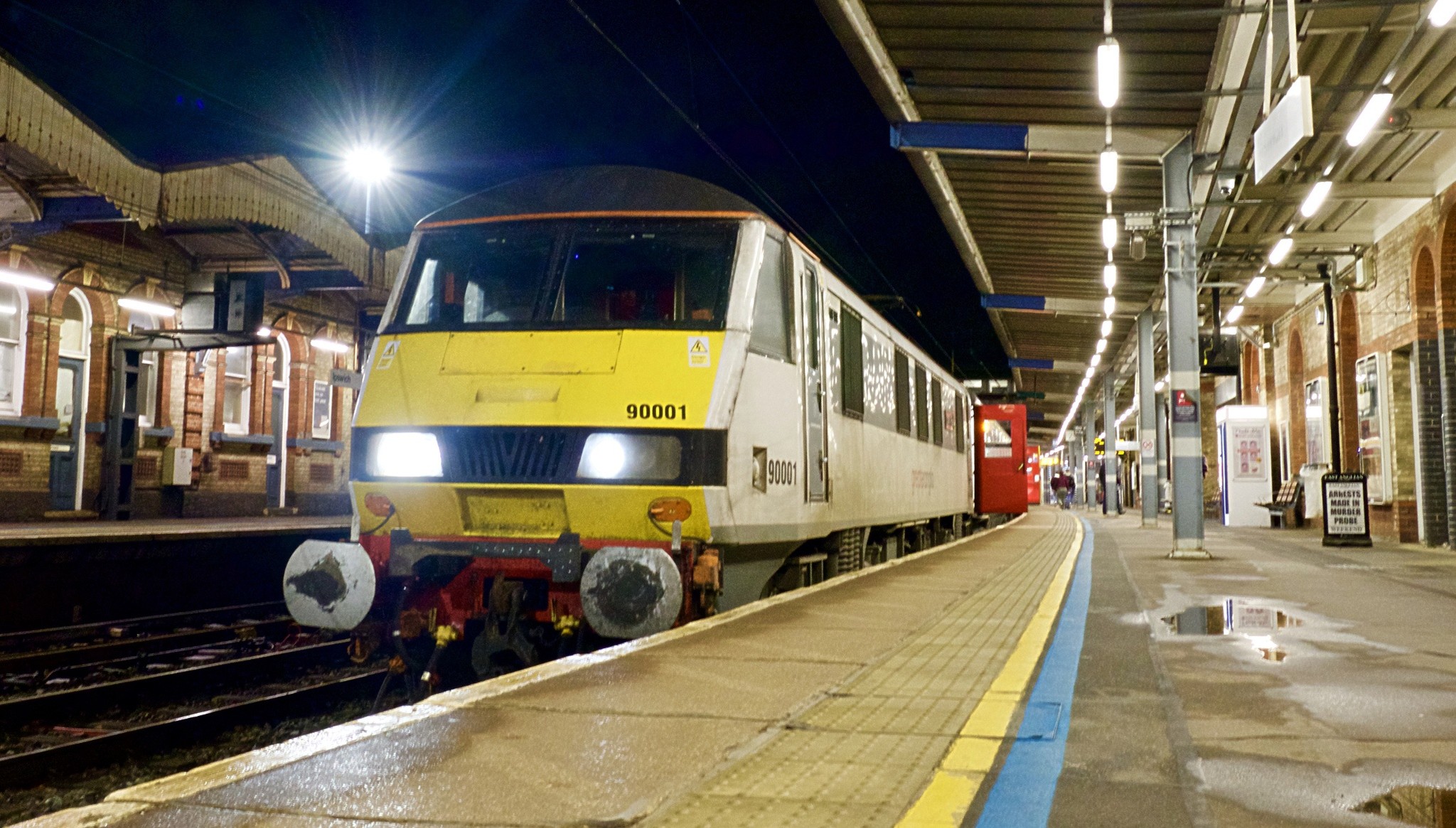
[1253, 477, 1302, 530]
[1203, 486, 1221, 518]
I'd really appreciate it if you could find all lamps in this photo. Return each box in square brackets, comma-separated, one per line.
[1096, 36, 1120, 354]
[1345, 86, 1393, 147]
[0, 267, 178, 316]
[1428, 0, 1455, 28]
[1224, 177, 1333, 324]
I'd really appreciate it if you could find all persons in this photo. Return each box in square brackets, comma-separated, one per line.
[1050, 469, 1075, 509]
[1099, 463, 1125, 514]
[1241, 441, 1257, 472]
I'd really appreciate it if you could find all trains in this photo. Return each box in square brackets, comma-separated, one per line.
[282, 161, 1042, 671]
